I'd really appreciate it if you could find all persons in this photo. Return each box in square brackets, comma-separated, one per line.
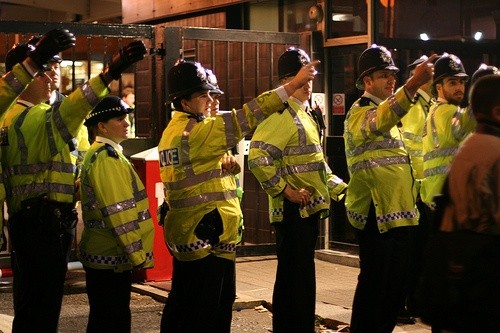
[342, 44, 500, 333]
[0, 26, 321, 333]
[247, 48, 352, 333]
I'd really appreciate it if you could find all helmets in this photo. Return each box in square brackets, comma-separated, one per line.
[355, 46, 400, 90]
[27, 37, 63, 64]
[6, 43, 36, 74]
[164, 61, 216, 107]
[469, 66, 500, 86]
[278, 47, 311, 81]
[430, 54, 468, 96]
[408, 58, 425, 75]
[205, 69, 224, 95]
[82, 95, 134, 126]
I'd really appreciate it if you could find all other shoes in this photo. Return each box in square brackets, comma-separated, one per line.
[397, 310, 416, 324]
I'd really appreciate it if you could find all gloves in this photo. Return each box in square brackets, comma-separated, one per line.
[29, 29, 76, 68]
[108, 40, 148, 79]
